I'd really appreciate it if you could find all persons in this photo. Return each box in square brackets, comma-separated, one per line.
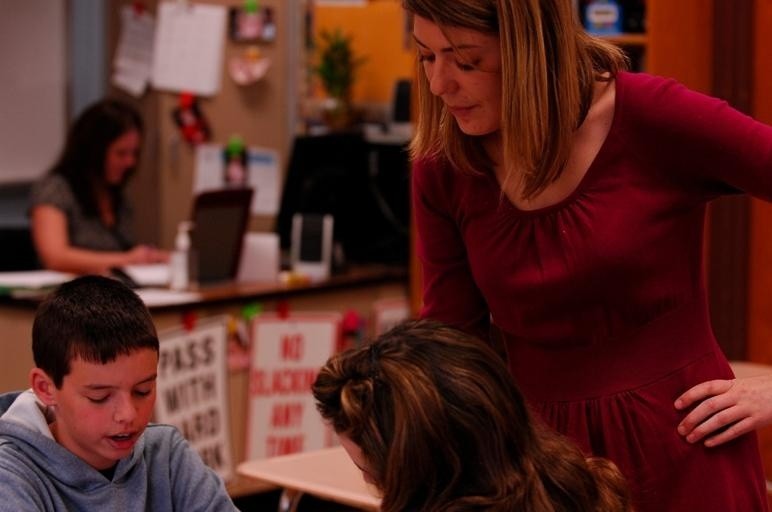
[310, 315, 635, 512]
[1, 272, 246, 512]
[403, 0, 772, 512]
[27, 94, 172, 276]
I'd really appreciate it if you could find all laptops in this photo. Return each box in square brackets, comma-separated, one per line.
[134, 185, 251, 286]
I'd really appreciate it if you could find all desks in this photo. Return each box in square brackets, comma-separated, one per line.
[235, 445, 388, 511]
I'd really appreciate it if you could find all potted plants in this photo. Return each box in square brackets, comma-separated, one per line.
[307, 28, 372, 130]
